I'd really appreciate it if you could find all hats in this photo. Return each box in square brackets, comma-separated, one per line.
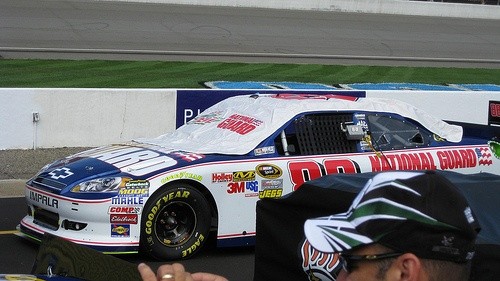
[303, 170, 482, 266]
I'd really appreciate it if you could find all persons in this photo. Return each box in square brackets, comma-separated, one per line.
[138, 167, 487, 281]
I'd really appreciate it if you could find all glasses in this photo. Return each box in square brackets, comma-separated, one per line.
[337, 251, 404, 274]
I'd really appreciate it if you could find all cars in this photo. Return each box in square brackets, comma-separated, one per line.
[13, 93, 500, 261]
